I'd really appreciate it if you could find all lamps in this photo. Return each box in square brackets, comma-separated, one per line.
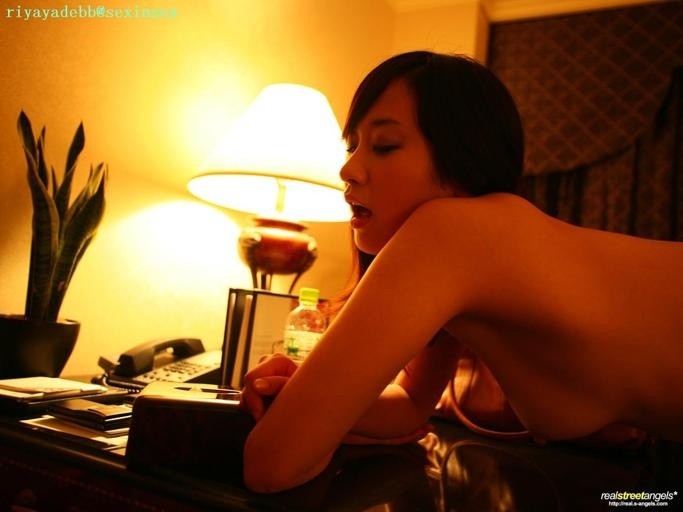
[187, 82, 356, 293]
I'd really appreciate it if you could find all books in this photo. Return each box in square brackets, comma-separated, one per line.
[0, 375, 132, 451]
[217, 286, 329, 392]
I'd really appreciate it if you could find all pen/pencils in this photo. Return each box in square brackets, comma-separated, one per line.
[174, 387, 239, 393]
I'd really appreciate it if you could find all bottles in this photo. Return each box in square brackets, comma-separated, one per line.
[283, 287, 325, 369]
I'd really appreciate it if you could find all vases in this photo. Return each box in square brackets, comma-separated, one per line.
[0, 108, 108, 379]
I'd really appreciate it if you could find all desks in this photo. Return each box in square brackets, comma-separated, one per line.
[0, 375, 437, 512]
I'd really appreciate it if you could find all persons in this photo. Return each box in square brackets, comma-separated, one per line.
[238, 48, 683, 492]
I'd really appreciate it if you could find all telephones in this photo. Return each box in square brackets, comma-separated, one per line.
[109, 336, 223, 391]
